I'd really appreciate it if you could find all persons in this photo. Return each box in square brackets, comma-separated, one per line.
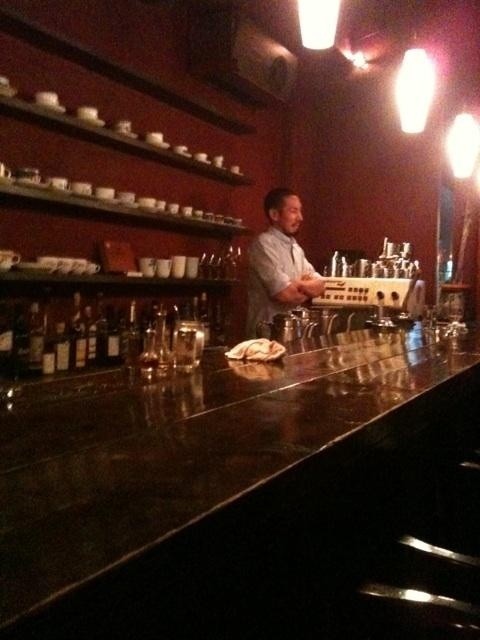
[246, 187, 326, 339]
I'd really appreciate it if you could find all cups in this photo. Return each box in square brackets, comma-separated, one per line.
[0, 251, 101, 275]
[139, 255, 198, 280]
[0, 90, 242, 227]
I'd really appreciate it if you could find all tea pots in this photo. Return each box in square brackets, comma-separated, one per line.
[256, 305, 356, 344]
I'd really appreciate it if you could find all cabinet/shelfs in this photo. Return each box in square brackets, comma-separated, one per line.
[0, 3, 256, 293]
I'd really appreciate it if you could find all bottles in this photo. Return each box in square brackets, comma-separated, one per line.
[0, 291, 225, 377]
